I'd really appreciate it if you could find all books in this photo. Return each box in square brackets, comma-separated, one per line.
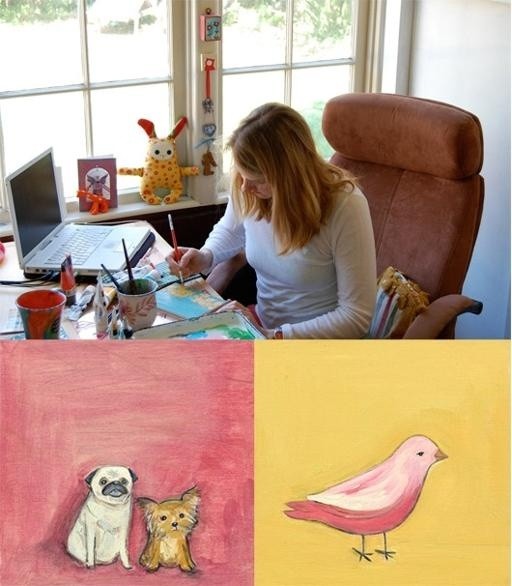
[152, 278, 231, 320]
[78, 154, 119, 211]
[142, 259, 200, 289]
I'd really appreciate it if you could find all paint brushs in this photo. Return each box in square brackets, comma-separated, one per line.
[168, 213, 185, 288]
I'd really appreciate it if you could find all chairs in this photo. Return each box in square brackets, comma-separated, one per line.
[320, 92, 484, 339]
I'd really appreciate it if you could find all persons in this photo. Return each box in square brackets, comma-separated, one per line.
[164, 101, 379, 342]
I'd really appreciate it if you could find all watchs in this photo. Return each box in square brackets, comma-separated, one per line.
[273, 324, 283, 339]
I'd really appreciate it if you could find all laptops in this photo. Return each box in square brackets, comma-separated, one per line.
[5, 146, 156, 280]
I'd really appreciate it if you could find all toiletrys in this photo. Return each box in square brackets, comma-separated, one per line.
[94, 271, 110, 340]
[60, 253, 78, 306]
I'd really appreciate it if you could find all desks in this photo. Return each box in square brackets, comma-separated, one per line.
[0, 219, 267, 339]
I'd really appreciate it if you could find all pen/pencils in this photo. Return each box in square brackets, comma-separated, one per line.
[101, 238, 138, 295]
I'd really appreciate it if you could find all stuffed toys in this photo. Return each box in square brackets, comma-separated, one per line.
[75, 188, 111, 214]
[118, 115, 201, 205]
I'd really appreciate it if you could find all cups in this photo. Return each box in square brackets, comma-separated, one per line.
[15, 289, 67, 340]
[115, 278, 159, 333]
